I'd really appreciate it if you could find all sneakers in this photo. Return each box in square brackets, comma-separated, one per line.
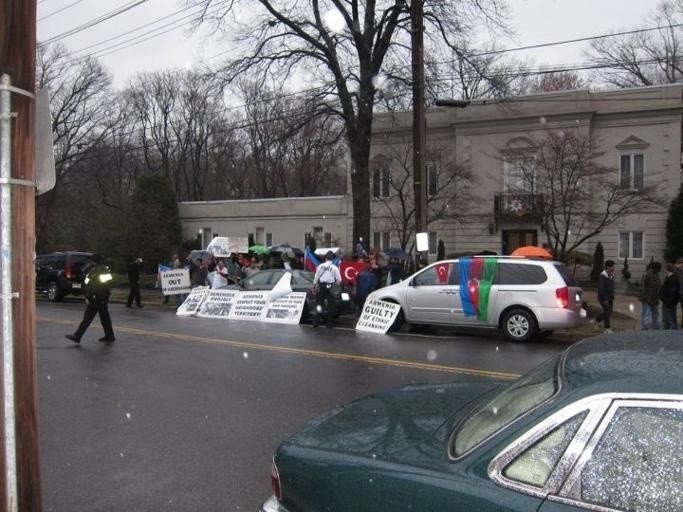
[99, 335, 115, 342]
[602, 328, 613, 335]
[590, 319, 600, 330]
[65, 334, 80, 344]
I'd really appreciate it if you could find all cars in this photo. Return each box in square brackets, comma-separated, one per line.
[257, 330, 682, 512]
[213, 268, 352, 323]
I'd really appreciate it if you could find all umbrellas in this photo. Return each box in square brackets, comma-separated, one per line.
[268, 244, 304, 257]
[248, 245, 269, 255]
[511, 246, 552, 258]
[383, 247, 408, 259]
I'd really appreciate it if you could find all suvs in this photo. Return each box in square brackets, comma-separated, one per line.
[35, 250, 99, 300]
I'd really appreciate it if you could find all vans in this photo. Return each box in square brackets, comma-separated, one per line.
[363, 255, 586, 342]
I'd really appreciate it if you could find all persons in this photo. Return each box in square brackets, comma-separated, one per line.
[126, 255, 143, 308]
[590, 260, 615, 334]
[639, 259, 683, 331]
[164, 251, 429, 328]
[65, 253, 115, 343]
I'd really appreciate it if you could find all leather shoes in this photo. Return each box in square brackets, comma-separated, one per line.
[126, 304, 144, 308]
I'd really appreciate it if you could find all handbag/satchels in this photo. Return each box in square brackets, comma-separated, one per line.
[311, 279, 321, 296]
